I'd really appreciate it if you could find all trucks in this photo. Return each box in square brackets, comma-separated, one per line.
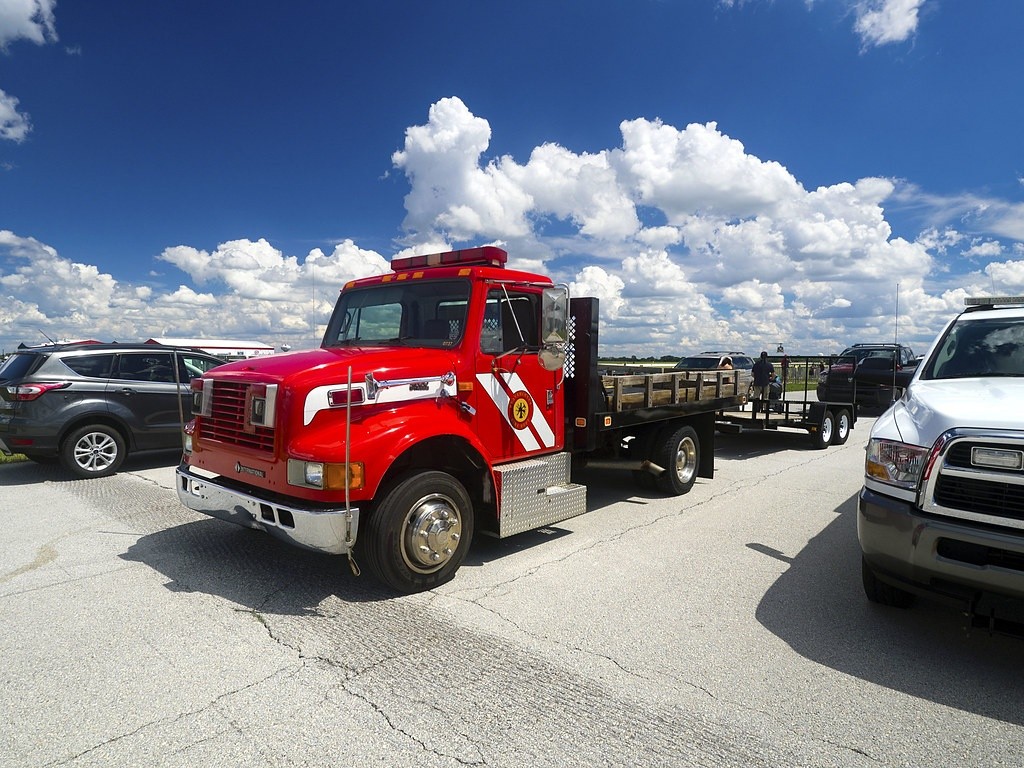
[174, 246, 757, 593]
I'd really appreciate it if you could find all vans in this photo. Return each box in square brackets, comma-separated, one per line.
[855, 291, 1024, 618]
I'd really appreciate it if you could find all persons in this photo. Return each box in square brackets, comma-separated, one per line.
[781, 354, 791, 378]
[809, 361, 824, 375]
[751, 351, 775, 412]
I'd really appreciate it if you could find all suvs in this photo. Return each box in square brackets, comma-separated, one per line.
[816, 342, 927, 411]
[0, 340, 234, 479]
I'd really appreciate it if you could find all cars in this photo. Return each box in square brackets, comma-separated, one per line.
[673, 349, 756, 374]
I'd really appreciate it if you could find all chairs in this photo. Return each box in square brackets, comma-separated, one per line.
[423, 320, 450, 339]
